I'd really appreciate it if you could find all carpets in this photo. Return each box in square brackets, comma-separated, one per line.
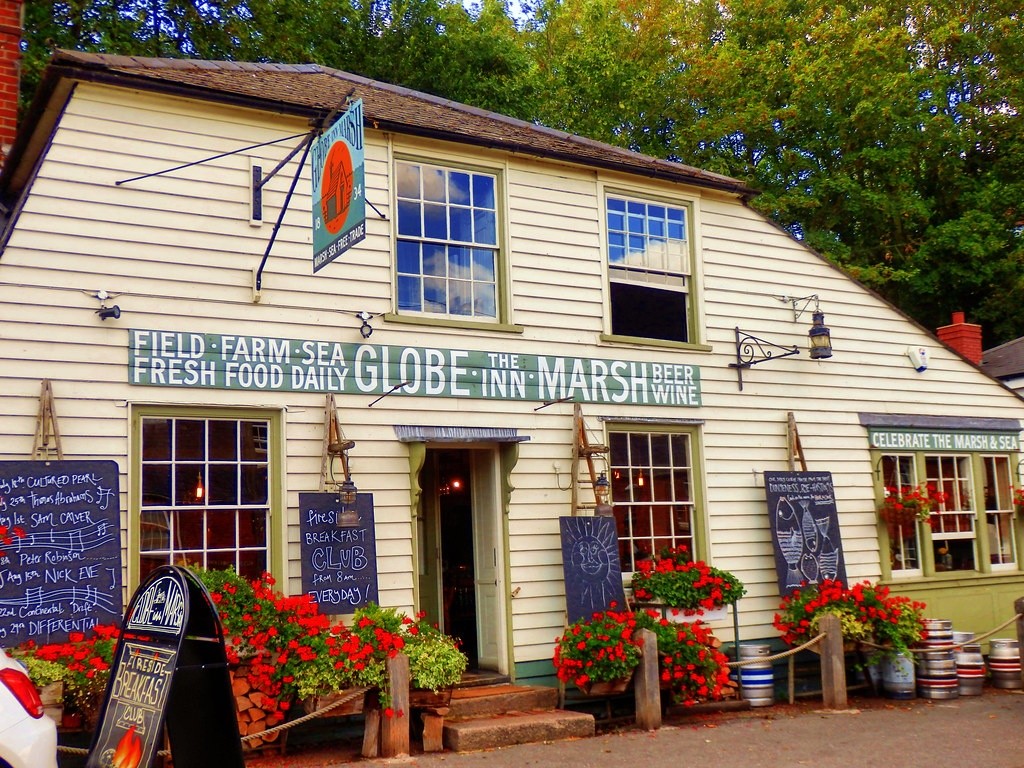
[451, 683, 534, 699]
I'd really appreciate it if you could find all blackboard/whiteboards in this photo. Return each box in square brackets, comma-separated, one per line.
[560, 516, 629, 625]
[0, 460, 124, 648]
[85, 565, 246, 768]
[298, 491, 380, 616]
[763, 470, 848, 596]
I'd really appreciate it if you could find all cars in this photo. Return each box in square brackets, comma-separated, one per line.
[0, 648, 57, 768]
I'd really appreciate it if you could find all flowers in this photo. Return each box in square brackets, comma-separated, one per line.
[1009, 484, 1024, 510]
[0, 526, 471, 720]
[771, 575, 934, 671]
[552, 542, 748, 709]
[877, 482, 948, 526]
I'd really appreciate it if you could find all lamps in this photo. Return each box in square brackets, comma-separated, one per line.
[356, 311, 373, 338]
[593, 452, 614, 518]
[192, 471, 205, 503]
[97, 289, 121, 322]
[791, 294, 833, 359]
[331, 452, 360, 528]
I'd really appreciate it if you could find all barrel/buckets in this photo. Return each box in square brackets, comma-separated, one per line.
[728, 645, 774, 705]
[915, 620, 1022, 699]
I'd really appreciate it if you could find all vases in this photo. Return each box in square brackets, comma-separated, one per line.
[408, 681, 455, 707]
[1018, 508, 1024, 517]
[305, 690, 363, 717]
[878, 507, 915, 525]
[580, 671, 633, 696]
